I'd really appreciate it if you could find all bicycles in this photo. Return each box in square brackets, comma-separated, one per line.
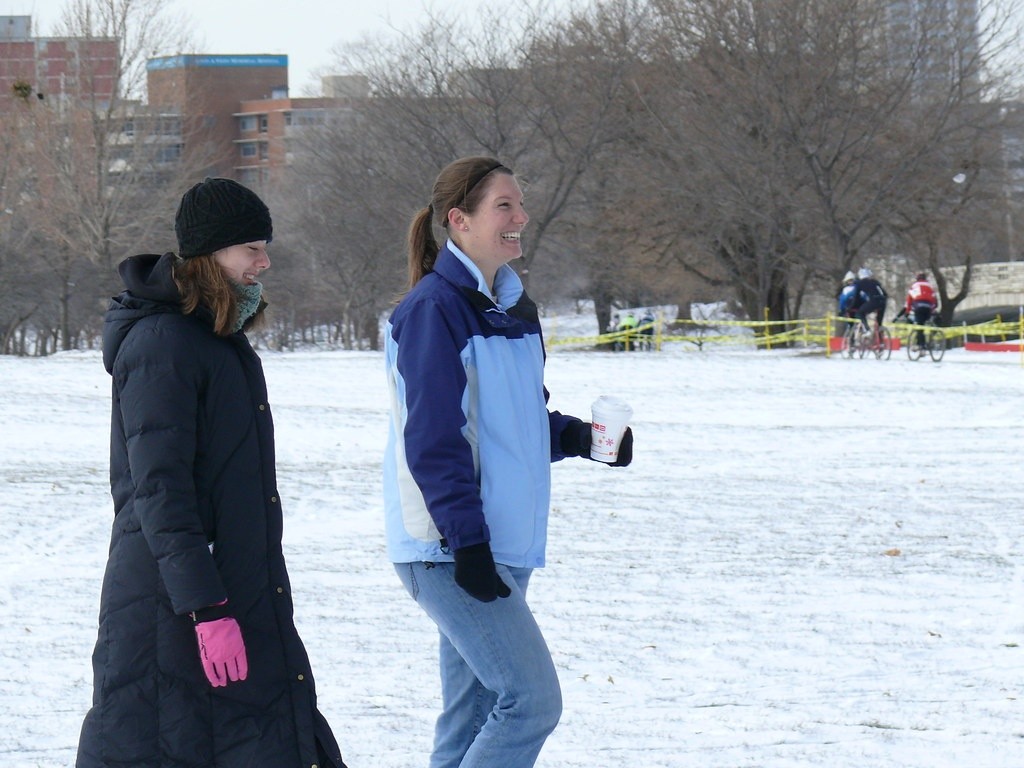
[839, 305, 892, 361]
[905, 311, 947, 362]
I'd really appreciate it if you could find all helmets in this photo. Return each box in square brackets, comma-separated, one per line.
[843, 271, 855, 282]
[858, 269, 871, 279]
[915, 272, 926, 281]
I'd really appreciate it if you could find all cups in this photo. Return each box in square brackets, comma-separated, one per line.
[590, 395, 631, 465]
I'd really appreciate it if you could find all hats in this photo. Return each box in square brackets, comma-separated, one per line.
[174, 178, 273, 259]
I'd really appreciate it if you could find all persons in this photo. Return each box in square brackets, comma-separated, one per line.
[382, 155, 634, 768]
[905, 273, 938, 356]
[72, 177, 352, 768]
[607, 309, 653, 351]
[835, 269, 889, 353]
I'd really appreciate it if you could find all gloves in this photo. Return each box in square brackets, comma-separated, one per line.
[454, 542, 512, 601]
[192, 598, 248, 688]
[564, 421, 634, 467]
